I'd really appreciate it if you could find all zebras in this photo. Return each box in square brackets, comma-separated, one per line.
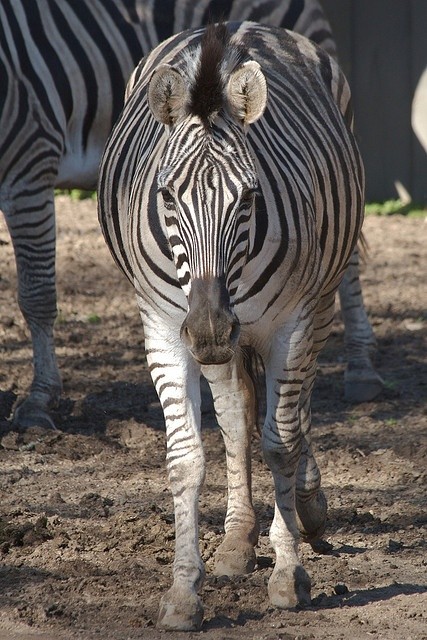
[1, 2, 384, 634]
